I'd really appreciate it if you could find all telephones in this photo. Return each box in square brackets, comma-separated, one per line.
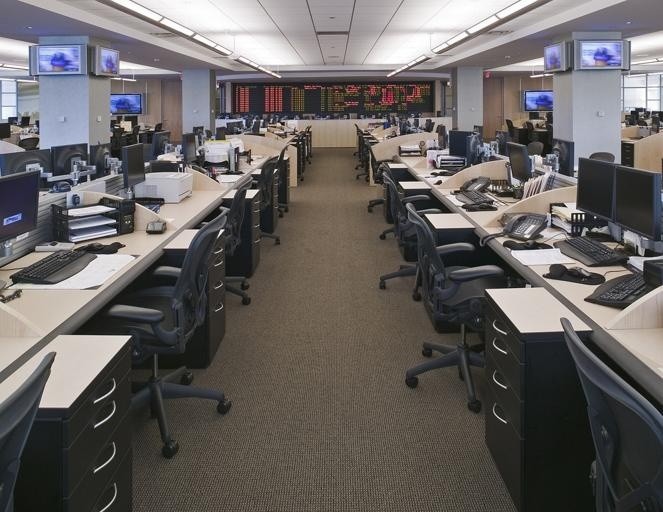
[460, 176, 490, 192]
[503, 213, 548, 240]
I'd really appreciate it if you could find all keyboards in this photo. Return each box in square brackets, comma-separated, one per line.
[10, 250, 97, 284]
[553, 237, 625, 267]
[584, 274, 654, 308]
[456, 190, 494, 205]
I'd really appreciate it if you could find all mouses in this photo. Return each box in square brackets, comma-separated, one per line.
[478, 203, 493, 209]
[87, 242, 102, 248]
[567, 267, 591, 277]
[526, 240, 538, 247]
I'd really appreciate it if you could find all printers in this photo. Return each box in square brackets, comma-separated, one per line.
[134, 172, 193, 202]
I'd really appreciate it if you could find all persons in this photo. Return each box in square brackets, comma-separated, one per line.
[114, 97, 130, 110]
[592, 45, 611, 62]
[534, 93, 549, 107]
[49, 51, 67, 67]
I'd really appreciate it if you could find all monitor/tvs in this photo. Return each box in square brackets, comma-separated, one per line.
[543, 40, 568, 73]
[523, 90, 554, 112]
[614, 164, 662, 244]
[573, 40, 630, 70]
[0, 170, 41, 243]
[387, 114, 575, 181]
[575, 156, 617, 223]
[109, 94, 141, 114]
[30, 44, 87, 74]
[93, 45, 120, 76]
[625, 108, 663, 137]
[0, 115, 282, 188]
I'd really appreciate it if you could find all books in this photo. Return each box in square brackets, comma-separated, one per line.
[548, 205, 588, 235]
[53, 204, 118, 243]
[398, 144, 421, 156]
[521, 170, 549, 202]
